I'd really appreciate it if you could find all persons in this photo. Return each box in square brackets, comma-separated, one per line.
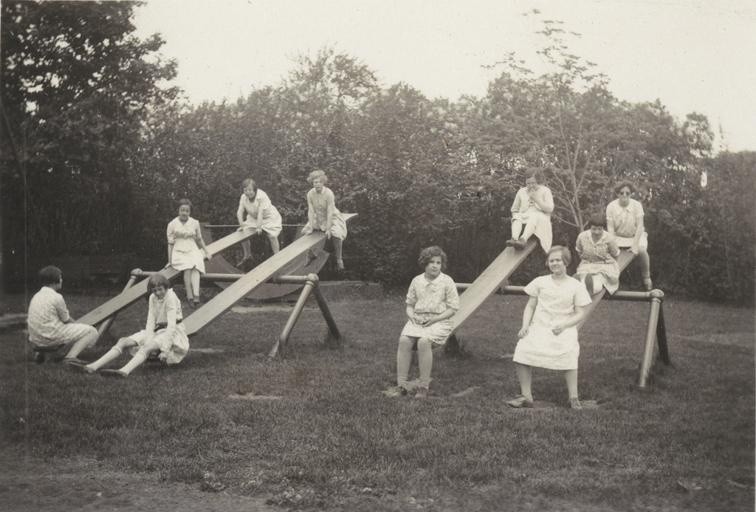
[386, 246, 459, 398]
[301, 170, 347, 272]
[576, 213, 620, 296]
[505, 168, 555, 253]
[27, 266, 99, 368]
[237, 179, 282, 266]
[607, 181, 652, 290]
[166, 200, 211, 308]
[507, 245, 592, 409]
[69, 274, 189, 376]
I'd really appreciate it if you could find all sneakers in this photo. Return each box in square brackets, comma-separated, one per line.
[507, 394, 536, 409]
[233, 253, 256, 268]
[383, 385, 429, 401]
[185, 294, 201, 306]
[567, 395, 582, 411]
[504, 235, 529, 251]
[36, 353, 93, 377]
[100, 368, 128, 381]
[641, 276, 654, 292]
[335, 257, 346, 273]
